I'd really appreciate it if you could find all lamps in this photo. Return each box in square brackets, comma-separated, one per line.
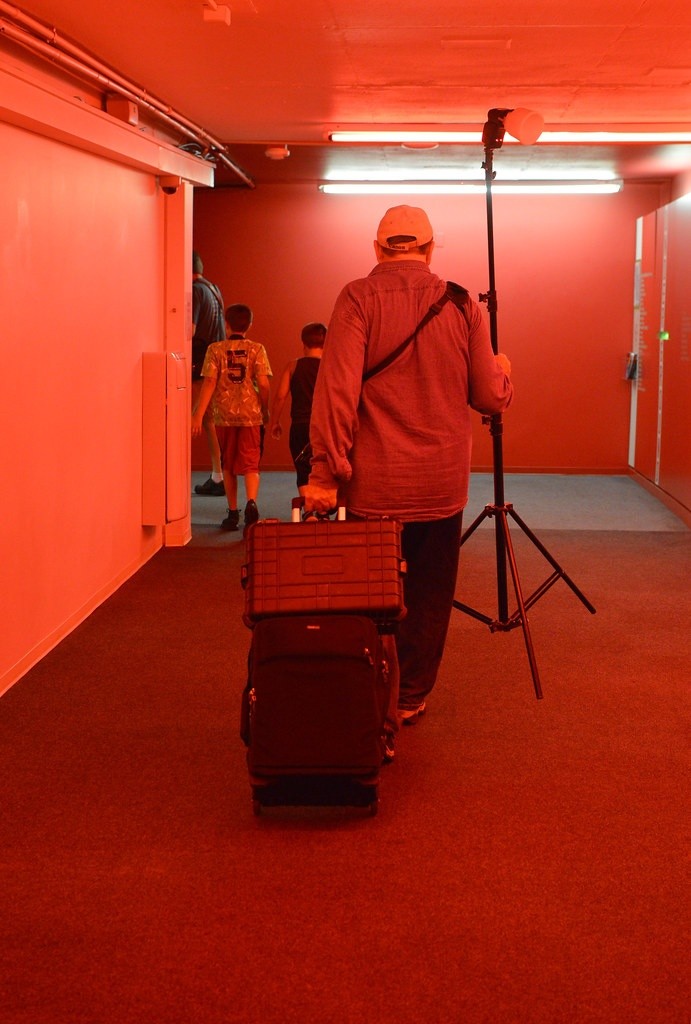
[328, 131, 691, 142]
[318, 179, 625, 194]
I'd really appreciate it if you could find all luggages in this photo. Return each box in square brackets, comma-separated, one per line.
[243, 498, 408, 816]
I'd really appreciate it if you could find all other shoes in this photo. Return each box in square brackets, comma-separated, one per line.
[244, 500, 259, 526]
[400, 702, 427, 722]
[221, 508, 240, 532]
[195, 474, 226, 496]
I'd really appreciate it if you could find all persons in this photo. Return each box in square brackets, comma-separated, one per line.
[305, 203, 513, 728]
[192, 304, 281, 531]
[271, 321, 330, 523]
[192, 250, 225, 496]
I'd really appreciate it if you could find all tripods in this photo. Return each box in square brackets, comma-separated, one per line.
[449, 151, 598, 702]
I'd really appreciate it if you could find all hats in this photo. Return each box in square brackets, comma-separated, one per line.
[377, 205, 433, 250]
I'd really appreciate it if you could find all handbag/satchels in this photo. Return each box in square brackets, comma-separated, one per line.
[193, 336, 214, 383]
